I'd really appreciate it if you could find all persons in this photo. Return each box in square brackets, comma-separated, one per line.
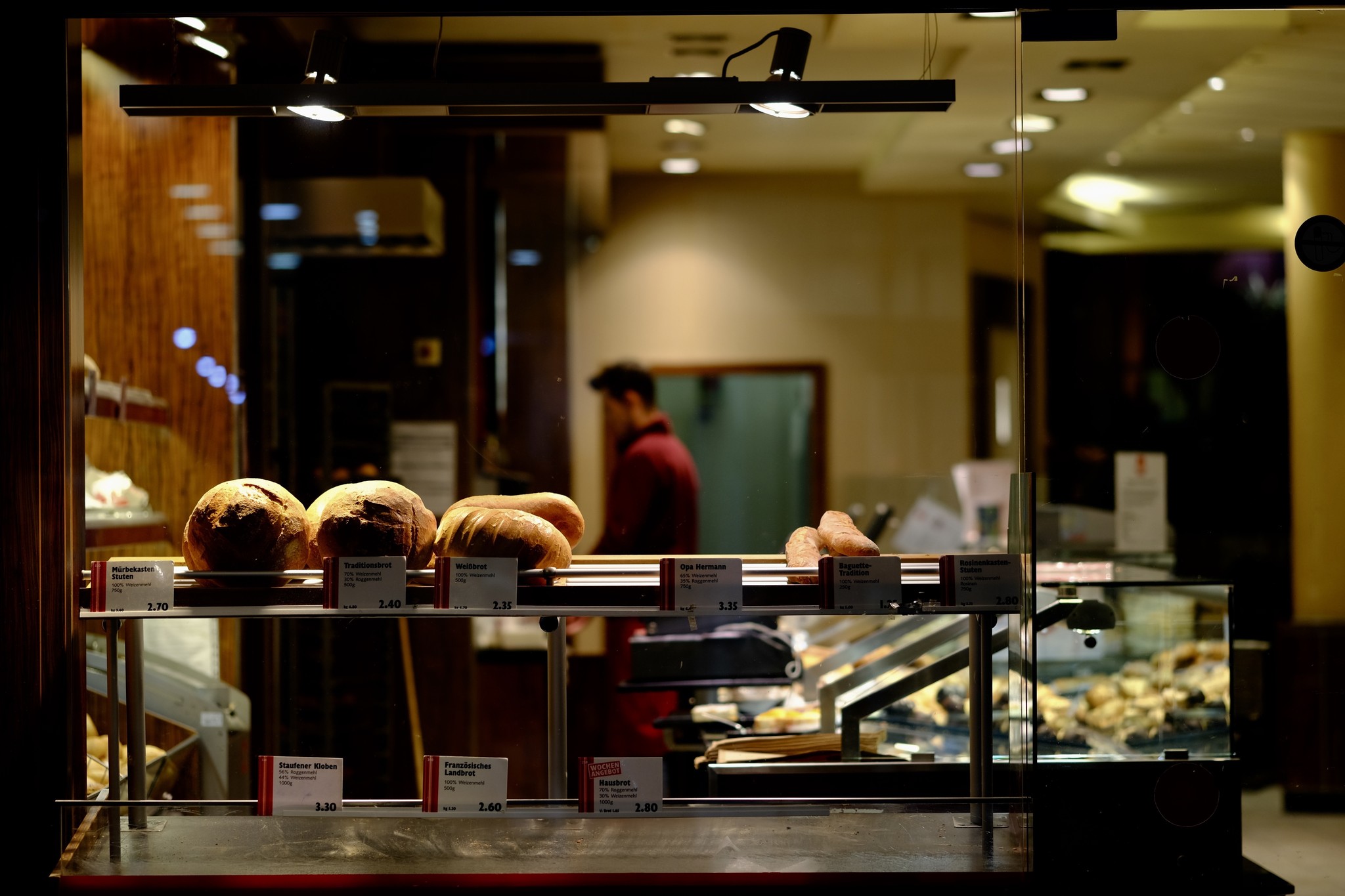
[565, 356, 704, 800]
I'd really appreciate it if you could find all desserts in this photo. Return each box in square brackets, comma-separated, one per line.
[1075, 640, 1229, 743]
[801, 644, 1071, 744]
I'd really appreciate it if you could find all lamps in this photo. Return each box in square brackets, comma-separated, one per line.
[286, 16, 360, 123]
[721, 26, 818, 120]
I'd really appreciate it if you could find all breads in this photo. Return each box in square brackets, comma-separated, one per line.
[181, 476, 584, 588]
[785, 508, 878, 586]
[752, 705, 821, 734]
